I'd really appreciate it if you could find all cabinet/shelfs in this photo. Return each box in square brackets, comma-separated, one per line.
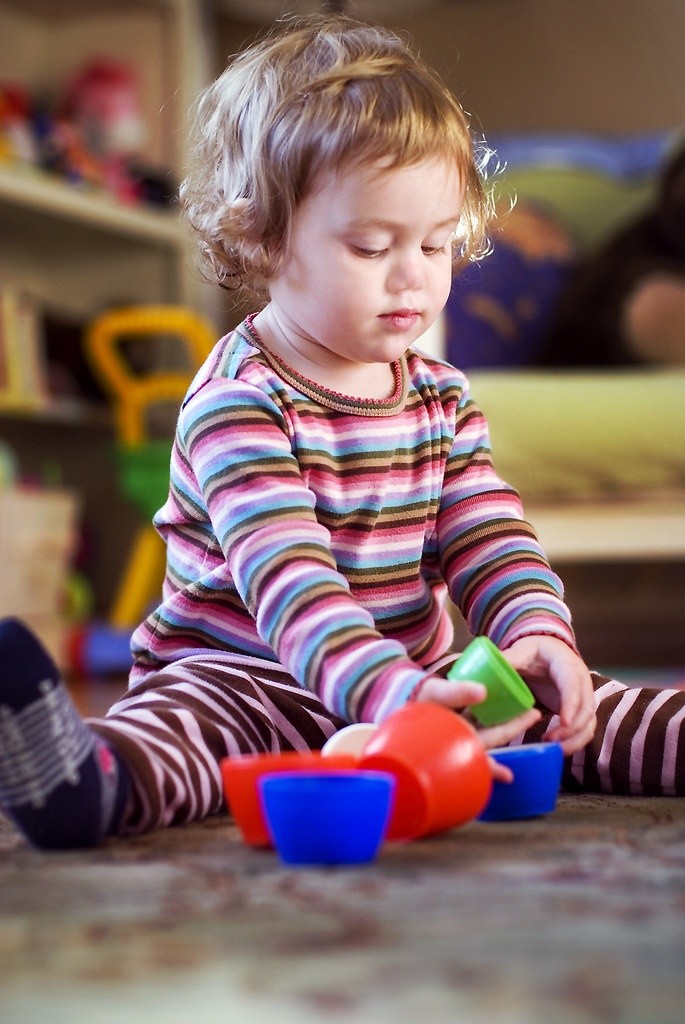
[0, 0, 224, 424]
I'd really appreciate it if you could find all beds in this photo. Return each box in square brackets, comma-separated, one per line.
[432, 133, 685, 571]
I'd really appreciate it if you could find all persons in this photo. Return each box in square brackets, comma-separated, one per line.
[529, 129, 685, 370]
[0, 20, 685, 852]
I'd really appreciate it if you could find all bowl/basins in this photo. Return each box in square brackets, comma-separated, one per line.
[362, 701, 492, 842]
[476, 740, 564, 820]
[258, 772, 393, 867]
[322, 723, 389, 757]
[223, 751, 318, 847]
[447, 636, 534, 727]
